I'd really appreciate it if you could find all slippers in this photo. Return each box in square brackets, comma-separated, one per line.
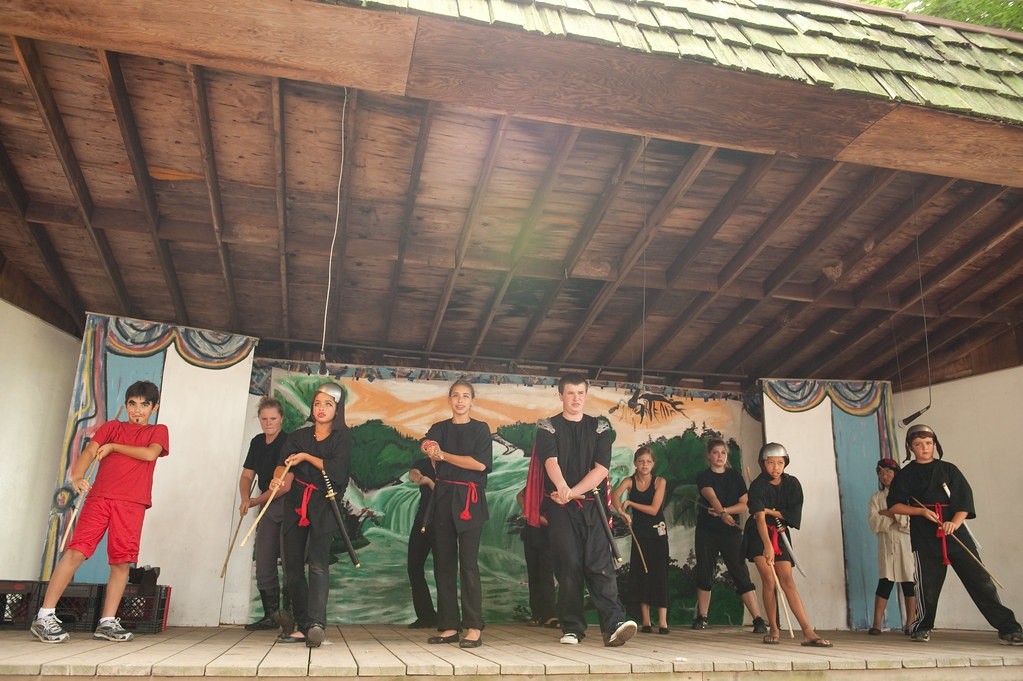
[763, 634, 779, 644]
[801, 638, 833, 648]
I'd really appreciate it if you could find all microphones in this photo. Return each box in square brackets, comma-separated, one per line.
[628, 380, 644, 409]
[898, 405, 931, 428]
[317, 351, 329, 378]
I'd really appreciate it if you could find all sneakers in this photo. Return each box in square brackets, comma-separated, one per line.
[93, 616, 134, 642]
[559, 633, 579, 644]
[915, 630, 930, 641]
[752, 617, 770, 633]
[605, 621, 637, 647]
[692, 615, 708, 630]
[30, 614, 70, 643]
[999, 631, 1023, 646]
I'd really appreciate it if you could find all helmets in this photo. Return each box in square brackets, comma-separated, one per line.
[762, 443, 788, 460]
[317, 382, 342, 403]
[906, 424, 934, 439]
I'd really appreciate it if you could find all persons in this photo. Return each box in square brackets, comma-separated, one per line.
[239, 397, 295, 638]
[421, 381, 493, 648]
[610, 448, 671, 633]
[269, 383, 354, 650]
[745, 443, 833, 647]
[693, 438, 769, 634]
[886, 423, 1023, 648]
[406, 456, 437, 630]
[515, 372, 637, 647]
[868, 457, 917, 636]
[30, 380, 169, 644]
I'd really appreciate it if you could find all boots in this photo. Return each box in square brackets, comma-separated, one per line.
[245, 587, 280, 630]
[282, 587, 298, 630]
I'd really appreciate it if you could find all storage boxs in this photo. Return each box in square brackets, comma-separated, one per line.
[0, 580, 45, 629]
[35, 580, 105, 632]
[99, 582, 171, 633]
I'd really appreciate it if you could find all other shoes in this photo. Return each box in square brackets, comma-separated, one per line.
[868, 628, 881, 635]
[659, 628, 670, 634]
[543, 620, 561, 628]
[278, 632, 306, 643]
[306, 621, 326, 648]
[459, 636, 482, 648]
[642, 626, 653, 633]
[528, 617, 544, 626]
[427, 632, 459, 644]
[905, 628, 912, 635]
[408, 618, 437, 629]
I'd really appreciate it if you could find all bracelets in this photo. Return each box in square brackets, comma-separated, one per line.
[719, 511, 726, 518]
[440, 452, 444, 461]
